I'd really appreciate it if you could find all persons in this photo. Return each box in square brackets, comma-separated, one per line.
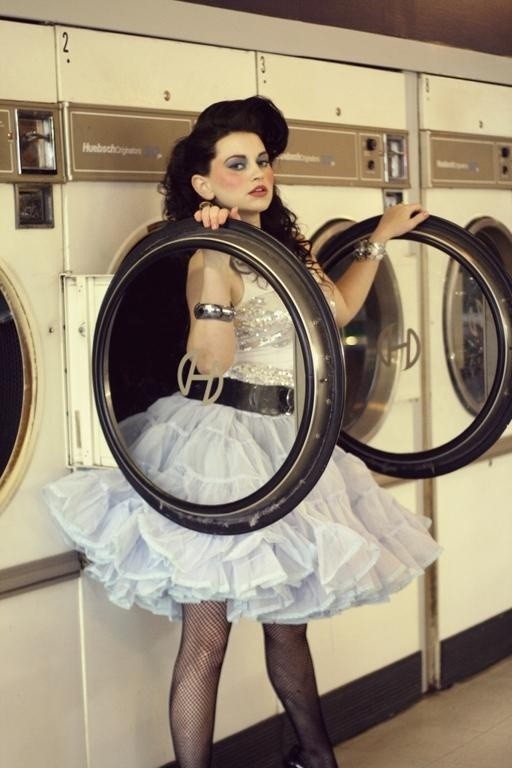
[125, 84, 432, 768]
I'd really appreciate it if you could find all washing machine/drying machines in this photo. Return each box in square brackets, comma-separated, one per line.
[417, 72, 512, 694]
[52, 24, 435, 768]
[0, 18, 92, 768]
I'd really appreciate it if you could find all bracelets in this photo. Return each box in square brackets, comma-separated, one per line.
[194, 302, 235, 325]
[356, 237, 387, 262]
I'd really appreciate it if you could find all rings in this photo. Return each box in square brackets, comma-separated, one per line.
[198, 202, 213, 210]
